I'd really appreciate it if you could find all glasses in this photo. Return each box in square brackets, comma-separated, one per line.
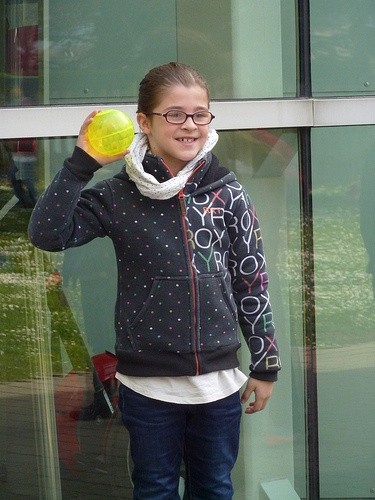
[146, 110, 215, 125]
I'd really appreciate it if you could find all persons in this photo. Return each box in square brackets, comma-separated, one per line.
[0, 142, 105, 500]
[26, 62, 282, 500]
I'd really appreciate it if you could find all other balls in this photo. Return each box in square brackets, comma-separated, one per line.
[87, 110, 134, 155]
